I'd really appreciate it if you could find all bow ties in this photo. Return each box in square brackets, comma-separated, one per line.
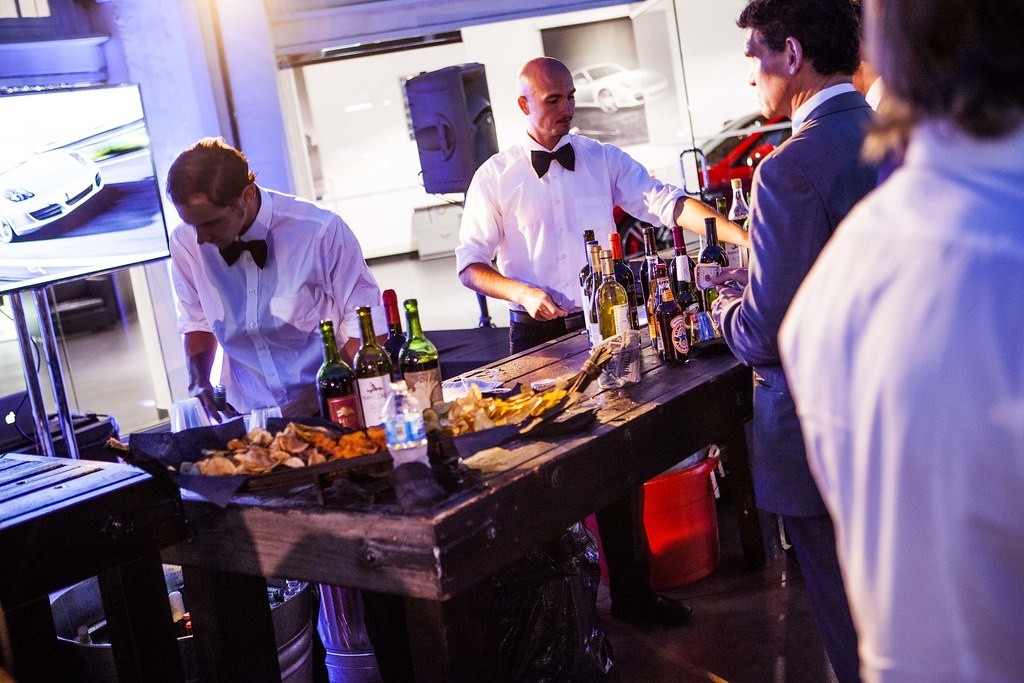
[218, 239, 268, 270]
[530, 140, 575, 180]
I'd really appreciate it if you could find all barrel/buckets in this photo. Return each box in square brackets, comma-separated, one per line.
[583, 442, 722, 592]
[50, 565, 313, 683]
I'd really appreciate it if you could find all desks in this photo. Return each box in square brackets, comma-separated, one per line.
[0, 452, 190, 683]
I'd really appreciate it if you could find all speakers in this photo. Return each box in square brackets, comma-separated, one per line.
[405, 62, 499, 195]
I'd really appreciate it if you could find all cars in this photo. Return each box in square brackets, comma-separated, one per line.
[0, 149, 104, 242]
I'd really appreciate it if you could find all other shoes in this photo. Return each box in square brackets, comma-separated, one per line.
[609, 591, 690, 627]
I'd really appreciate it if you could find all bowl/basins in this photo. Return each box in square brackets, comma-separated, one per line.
[589, 343, 642, 389]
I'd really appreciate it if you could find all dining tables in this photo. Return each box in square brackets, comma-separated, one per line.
[159, 327, 775, 683]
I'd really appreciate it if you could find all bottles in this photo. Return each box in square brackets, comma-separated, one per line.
[209, 383, 237, 424]
[578, 178, 751, 368]
[77, 577, 301, 645]
[315, 288, 457, 489]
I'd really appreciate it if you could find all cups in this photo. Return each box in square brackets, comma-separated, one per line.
[248, 405, 282, 434]
[170, 396, 212, 497]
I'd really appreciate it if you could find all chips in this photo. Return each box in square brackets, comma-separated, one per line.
[181, 423, 387, 474]
[422, 383, 567, 436]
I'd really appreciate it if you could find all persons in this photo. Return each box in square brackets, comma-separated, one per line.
[705, 0, 1024, 683]
[453, 57, 751, 625]
[167, 136, 380, 425]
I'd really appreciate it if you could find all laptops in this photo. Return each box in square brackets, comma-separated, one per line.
[0, 391, 35, 452]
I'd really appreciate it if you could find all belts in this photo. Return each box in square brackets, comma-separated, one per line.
[510, 310, 585, 330]
